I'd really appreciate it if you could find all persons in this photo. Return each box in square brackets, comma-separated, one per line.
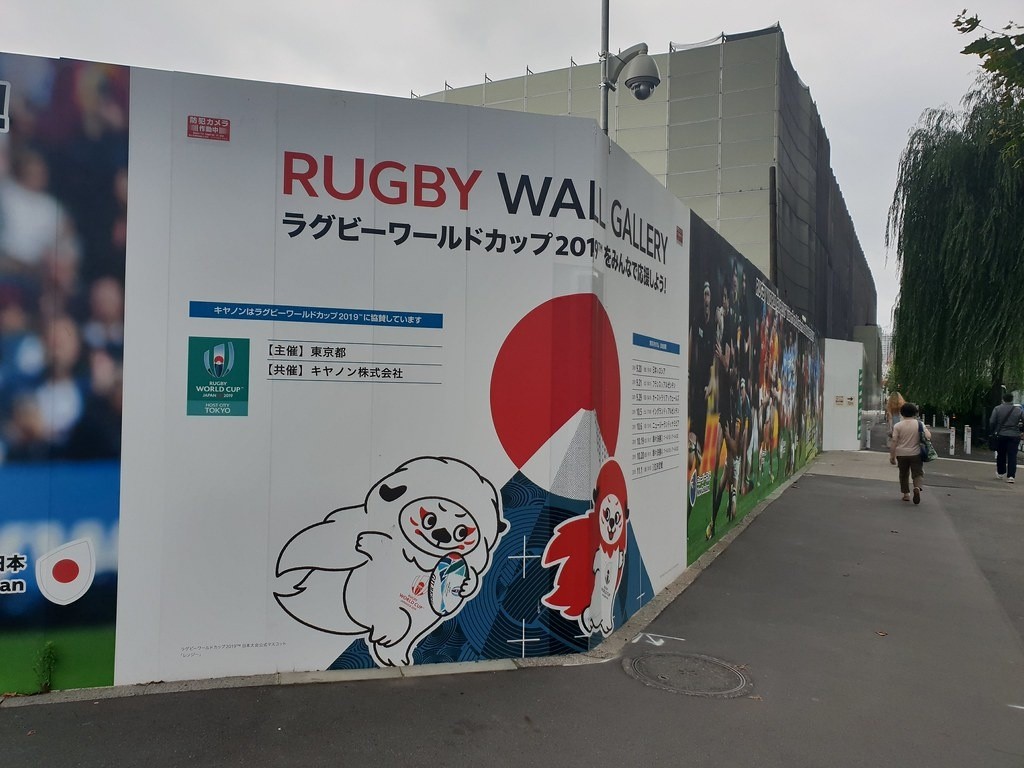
[688, 260, 823, 539]
[887, 392, 906, 434]
[990, 394, 1024, 482]
[889, 403, 931, 504]
[0, 64, 128, 458]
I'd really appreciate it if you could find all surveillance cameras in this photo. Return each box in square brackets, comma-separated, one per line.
[624, 55, 662, 101]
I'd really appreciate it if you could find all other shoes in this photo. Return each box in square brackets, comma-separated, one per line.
[913, 487, 920, 504]
[996, 471, 1005, 479]
[769, 471, 774, 480]
[705, 523, 715, 540]
[903, 496, 911, 500]
[1006, 477, 1015, 482]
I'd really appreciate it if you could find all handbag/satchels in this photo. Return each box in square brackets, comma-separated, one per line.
[917, 420, 938, 462]
[988, 435, 998, 452]
